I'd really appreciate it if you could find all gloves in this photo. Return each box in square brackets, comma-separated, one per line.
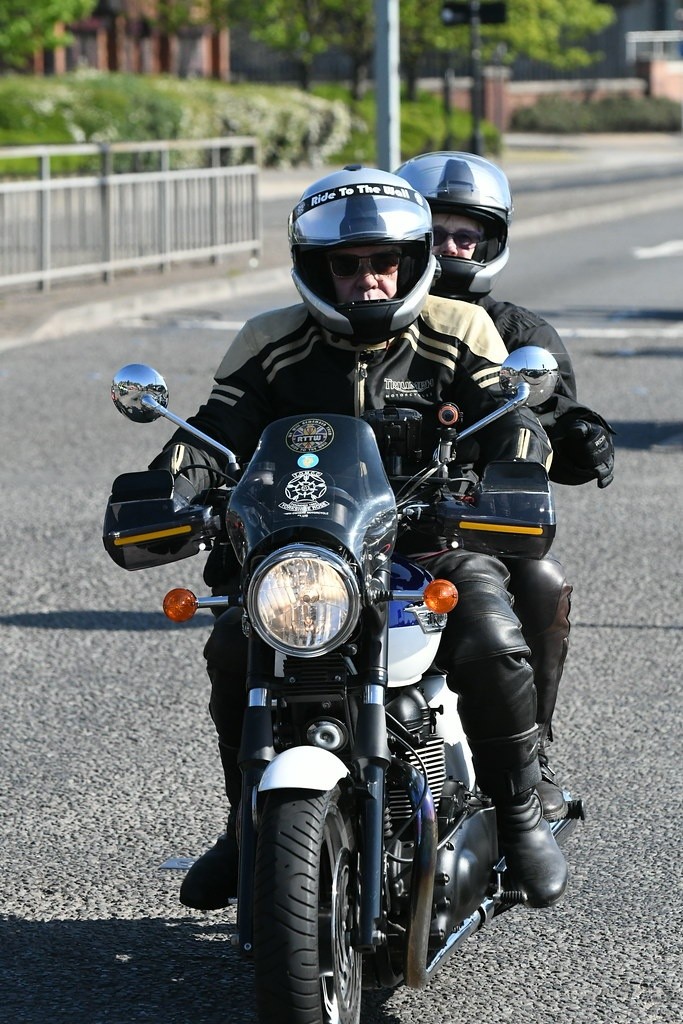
[476, 429, 554, 546]
[562, 407, 617, 488]
[117, 441, 216, 555]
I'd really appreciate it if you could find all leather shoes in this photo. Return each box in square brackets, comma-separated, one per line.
[498, 790, 571, 911]
[179, 835, 238, 910]
[534, 746, 569, 822]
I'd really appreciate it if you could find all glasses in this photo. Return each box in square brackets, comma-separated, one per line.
[328, 250, 402, 279]
[433, 225, 485, 249]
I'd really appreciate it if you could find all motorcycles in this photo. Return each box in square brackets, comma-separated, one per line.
[107, 342, 585, 1023]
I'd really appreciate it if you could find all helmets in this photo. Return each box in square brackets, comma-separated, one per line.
[286, 165, 438, 340]
[391, 151, 514, 299]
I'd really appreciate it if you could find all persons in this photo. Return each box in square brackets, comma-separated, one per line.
[387, 151, 615, 823]
[144, 162, 574, 914]
